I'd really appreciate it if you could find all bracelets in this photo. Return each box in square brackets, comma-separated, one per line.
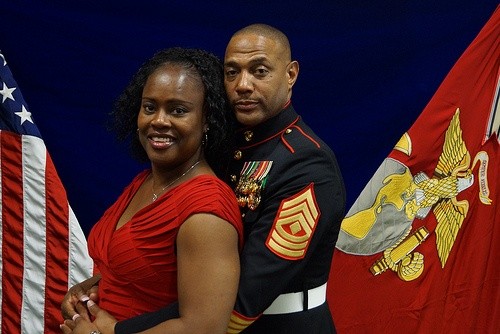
[90, 330, 100, 334]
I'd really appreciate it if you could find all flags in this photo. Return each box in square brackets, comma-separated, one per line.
[0, 51, 96, 334]
[326, 3, 500, 334]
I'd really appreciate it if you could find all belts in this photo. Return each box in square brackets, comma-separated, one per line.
[262, 282, 326, 315]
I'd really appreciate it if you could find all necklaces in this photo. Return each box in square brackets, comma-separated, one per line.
[152, 162, 200, 202]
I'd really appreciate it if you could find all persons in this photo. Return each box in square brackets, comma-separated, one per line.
[60, 48, 244, 334]
[75, 23, 345, 334]
